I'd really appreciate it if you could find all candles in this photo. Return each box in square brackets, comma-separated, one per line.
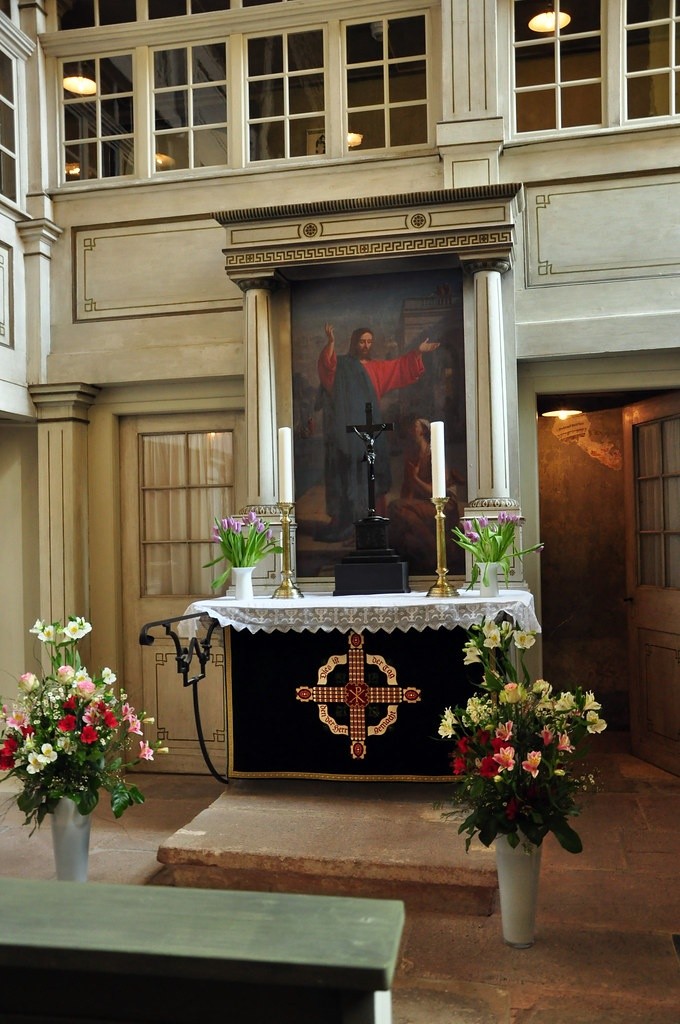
[278, 426, 292, 501]
[430, 422, 446, 498]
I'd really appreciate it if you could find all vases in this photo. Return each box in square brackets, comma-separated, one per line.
[475, 562, 500, 598]
[232, 567, 255, 600]
[50, 797, 90, 879]
[496, 827, 544, 949]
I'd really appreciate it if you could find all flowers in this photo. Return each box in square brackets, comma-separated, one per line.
[0, 617, 170, 827]
[204, 513, 285, 587]
[433, 616, 609, 853]
[450, 510, 544, 592]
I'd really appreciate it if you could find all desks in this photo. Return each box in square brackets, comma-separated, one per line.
[0, 879, 405, 1024]
